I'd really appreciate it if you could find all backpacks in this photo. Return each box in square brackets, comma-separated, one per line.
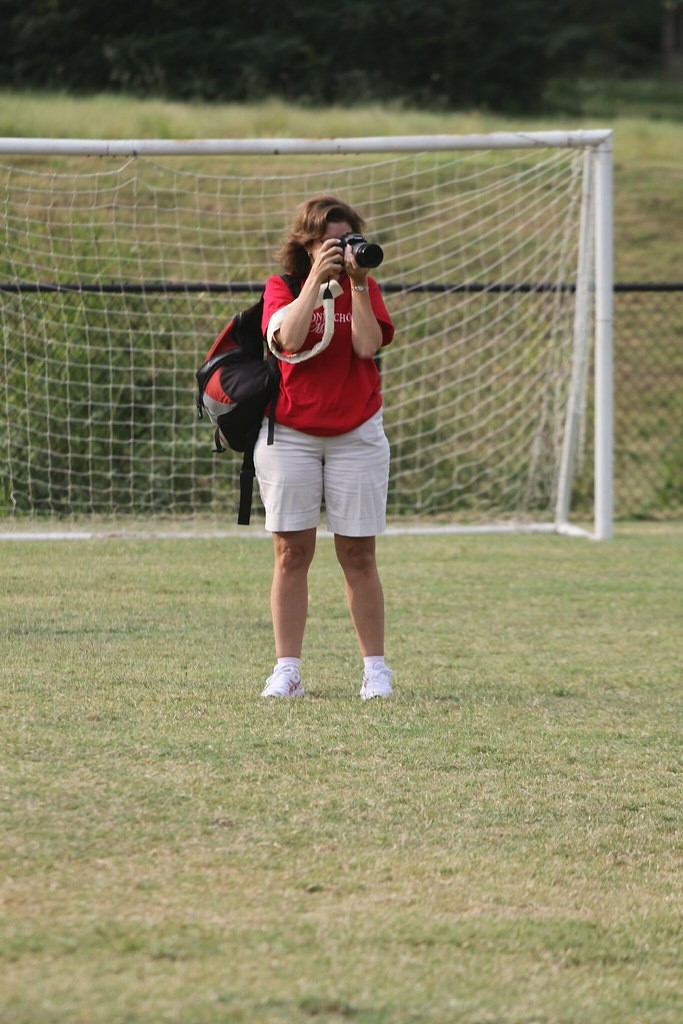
[194, 272, 305, 457]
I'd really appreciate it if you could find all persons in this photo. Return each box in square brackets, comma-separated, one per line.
[251, 195, 400, 701]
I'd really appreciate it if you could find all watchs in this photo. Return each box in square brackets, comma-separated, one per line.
[350, 284, 369, 292]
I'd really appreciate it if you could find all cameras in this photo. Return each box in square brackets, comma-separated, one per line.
[333, 232, 384, 268]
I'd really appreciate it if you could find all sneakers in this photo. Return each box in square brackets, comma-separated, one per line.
[260, 662, 305, 703]
[358, 660, 394, 704]
